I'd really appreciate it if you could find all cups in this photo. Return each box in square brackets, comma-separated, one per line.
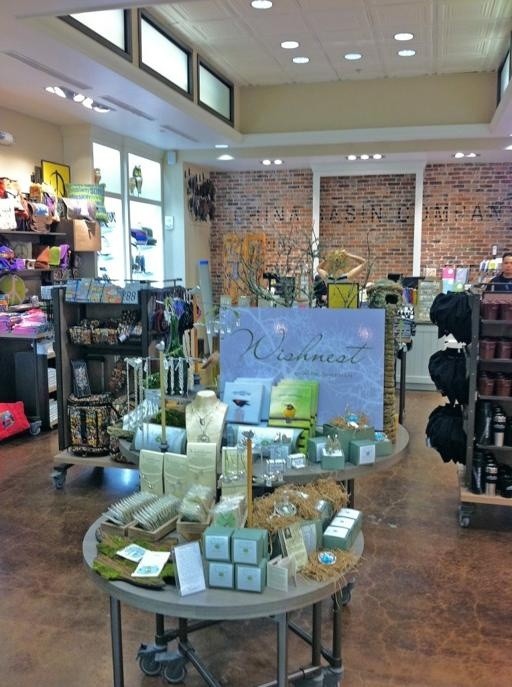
[476, 297, 511, 396]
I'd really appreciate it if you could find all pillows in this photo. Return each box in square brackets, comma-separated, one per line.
[0, 401, 30, 440]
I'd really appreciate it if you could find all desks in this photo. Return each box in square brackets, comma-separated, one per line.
[82, 507, 365, 687]
[394, 339, 412, 424]
[119, 421, 409, 605]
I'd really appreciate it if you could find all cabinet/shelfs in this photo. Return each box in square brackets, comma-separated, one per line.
[51, 286, 193, 490]
[458, 282, 512, 532]
[0, 230, 67, 438]
[396, 321, 445, 392]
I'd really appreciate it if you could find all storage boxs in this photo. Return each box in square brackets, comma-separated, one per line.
[56, 219, 100, 252]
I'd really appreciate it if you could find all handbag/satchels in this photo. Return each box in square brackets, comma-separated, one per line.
[67, 392, 112, 457]
[68, 309, 141, 347]
[107, 391, 143, 464]
[0, 176, 67, 234]
[16, 244, 72, 271]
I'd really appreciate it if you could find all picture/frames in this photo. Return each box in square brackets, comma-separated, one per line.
[41, 159, 70, 197]
[327, 283, 359, 309]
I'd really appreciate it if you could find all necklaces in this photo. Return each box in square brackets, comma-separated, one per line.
[125, 356, 289, 491]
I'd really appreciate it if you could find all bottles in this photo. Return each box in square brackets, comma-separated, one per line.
[472, 399, 511, 499]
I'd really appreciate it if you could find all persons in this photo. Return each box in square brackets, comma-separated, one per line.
[484, 252, 512, 293]
[316, 247, 366, 287]
[315, 274, 328, 306]
